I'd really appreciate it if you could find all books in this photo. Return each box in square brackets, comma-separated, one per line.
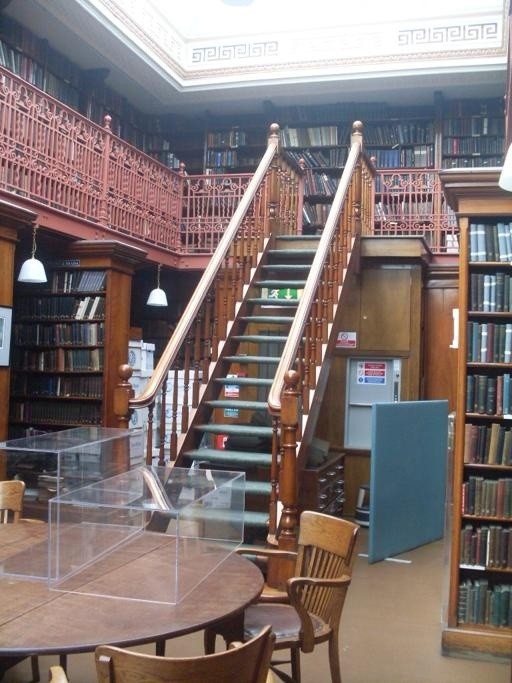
[1, 14, 180, 171]
[464, 221, 511, 468]
[206, 128, 247, 206]
[273, 102, 433, 226]
[10, 267, 106, 483]
[442, 97, 505, 168]
[455, 475, 512, 629]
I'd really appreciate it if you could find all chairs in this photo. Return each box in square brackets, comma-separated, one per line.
[93, 623, 276, 682]
[205, 511, 359, 682]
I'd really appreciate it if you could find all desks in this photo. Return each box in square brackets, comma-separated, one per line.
[0, 518, 265, 681]
